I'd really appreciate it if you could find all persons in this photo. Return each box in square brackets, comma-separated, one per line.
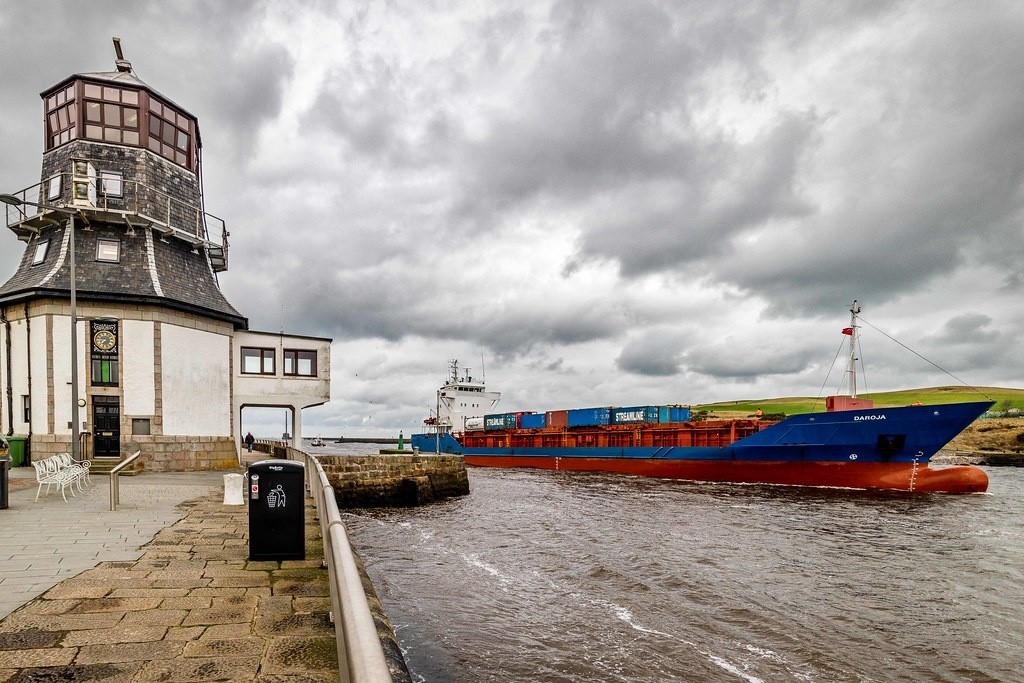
[245, 431, 255, 453]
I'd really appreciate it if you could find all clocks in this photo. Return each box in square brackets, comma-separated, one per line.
[94, 330, 115, 350]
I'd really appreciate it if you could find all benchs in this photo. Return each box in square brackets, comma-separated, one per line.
[31, 453, 92, 503]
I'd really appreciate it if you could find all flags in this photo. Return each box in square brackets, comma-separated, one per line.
[842, 328, 853, 336]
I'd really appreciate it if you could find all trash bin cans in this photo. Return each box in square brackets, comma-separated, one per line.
[248, 458, 306, 561]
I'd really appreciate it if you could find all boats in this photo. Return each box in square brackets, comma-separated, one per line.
[311, 438, 326, 446]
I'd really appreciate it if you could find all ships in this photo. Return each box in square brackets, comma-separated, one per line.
[410, 297, 998, 494]
